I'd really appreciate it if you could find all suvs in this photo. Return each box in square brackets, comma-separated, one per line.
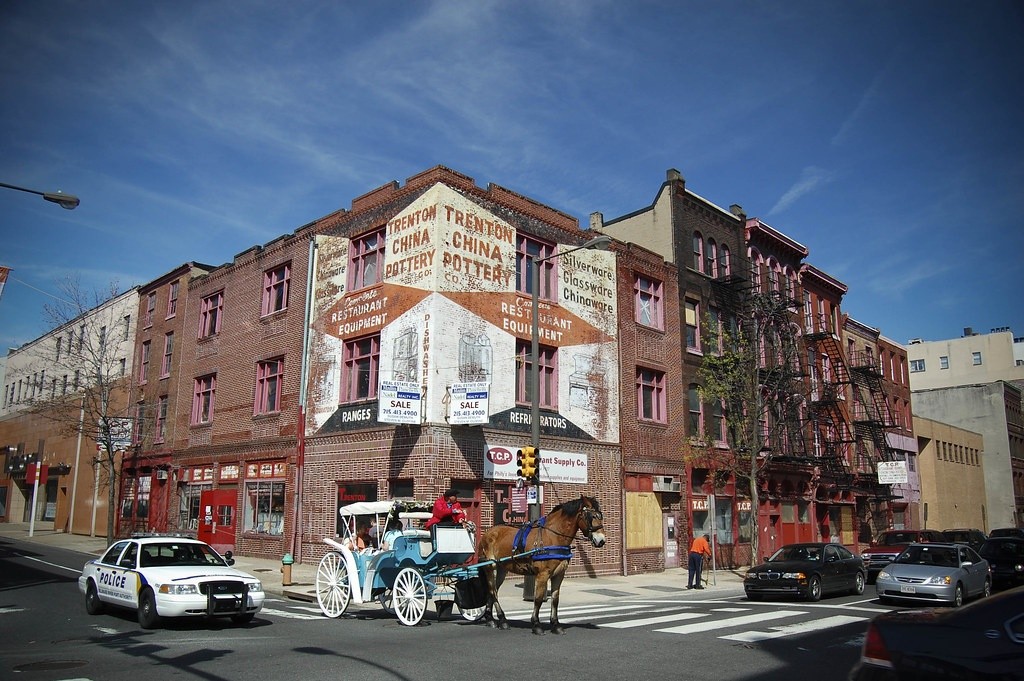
[940, 527, 988, 555]
[860, 528, 947, 579]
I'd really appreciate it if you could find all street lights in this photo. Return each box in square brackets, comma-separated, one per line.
[523, 233, 615, 602]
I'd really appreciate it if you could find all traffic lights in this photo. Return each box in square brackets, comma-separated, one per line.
[525, 446, 539, 478]
[516, 446, 527, 477]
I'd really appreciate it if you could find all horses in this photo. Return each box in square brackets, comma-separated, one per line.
[477, 494, 606, 634]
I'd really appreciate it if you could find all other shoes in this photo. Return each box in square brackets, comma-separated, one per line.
[687, 585, 695, 589]
[695, 585, 704, 589]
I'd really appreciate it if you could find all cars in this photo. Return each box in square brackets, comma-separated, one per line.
[985, 527, 1024, 540]
[976, 537, 1024, 593]
[742, 541, 868, 601]
[77, 531, 265, 629]
[875, 542, 993, 608]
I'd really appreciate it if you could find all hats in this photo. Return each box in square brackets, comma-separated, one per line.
[704, 535, 710, 542]
[444, 489, 460, 497]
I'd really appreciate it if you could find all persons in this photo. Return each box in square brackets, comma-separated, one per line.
[687, 535, 712, 590]
[941, 551, 955, 567]
[342, 517, 404, 553]
[425, 489, 465, 530]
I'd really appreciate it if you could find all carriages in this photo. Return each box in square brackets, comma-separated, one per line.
[313, 491, 606, 636]
